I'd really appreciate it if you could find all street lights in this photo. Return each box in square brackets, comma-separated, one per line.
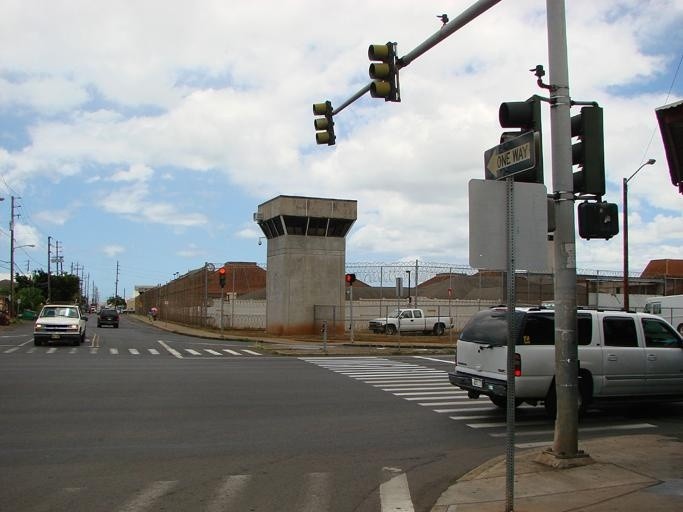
[406, 270, 411, 304]
[11, 244, 36, 318]
[622, 159, 656, 310]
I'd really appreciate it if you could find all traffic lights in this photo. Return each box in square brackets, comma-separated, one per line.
[219, 268, 226, 285]
[312, 100, 337, 147]
[346, 274, 356, 283]
[571, 106, 606, 195]
[499, 94, 541, 144]
[367, 42, 402, 103]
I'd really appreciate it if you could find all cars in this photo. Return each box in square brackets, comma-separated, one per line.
[97, 308, 119, 328]
[122, 307, 135, 314]
[32, 304, 87, 346]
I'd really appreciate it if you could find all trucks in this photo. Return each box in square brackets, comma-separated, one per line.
[643, 295, 683, 336]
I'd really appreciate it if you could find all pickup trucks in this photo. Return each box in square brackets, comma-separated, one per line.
[368, 309, 455, 336]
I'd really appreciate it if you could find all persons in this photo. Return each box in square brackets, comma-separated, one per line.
[152, 309, 157, 321]
[148, 310, 152, 320]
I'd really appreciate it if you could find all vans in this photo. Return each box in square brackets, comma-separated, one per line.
[448, 307, 683, 420]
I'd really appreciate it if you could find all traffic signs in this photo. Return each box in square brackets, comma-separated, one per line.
[484, 130, 535, 181]
